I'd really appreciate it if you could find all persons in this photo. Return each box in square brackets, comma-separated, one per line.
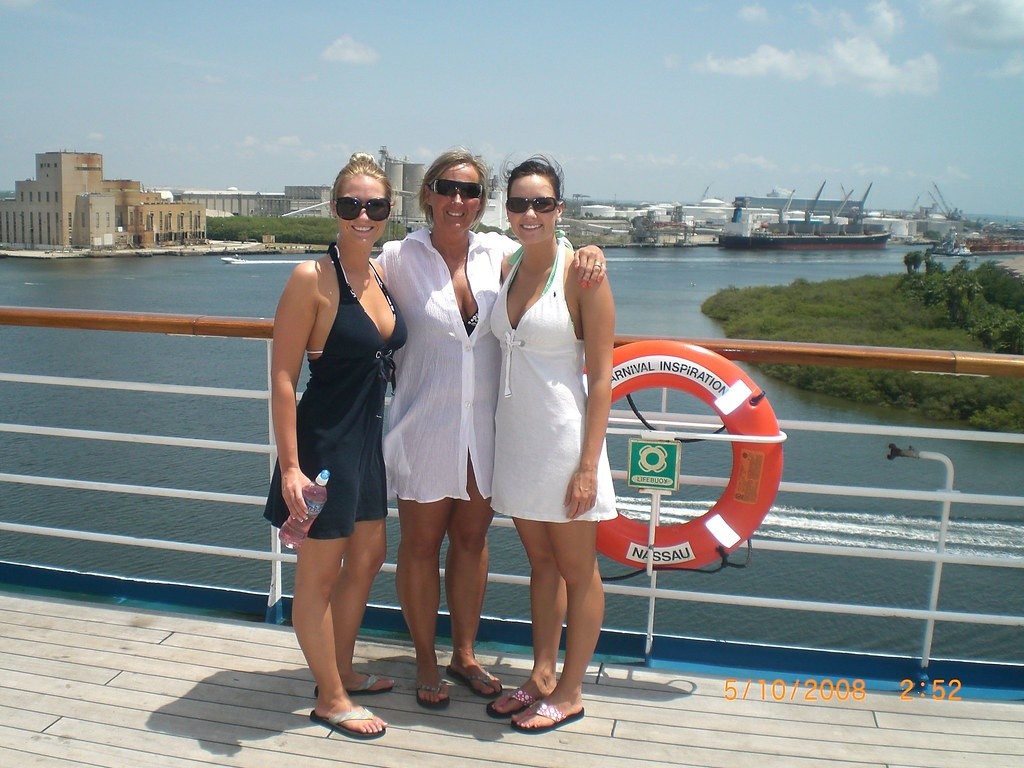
[263, 152, 407, 738]
[378, 151, 606, 707]
[486, 157, 619, 732]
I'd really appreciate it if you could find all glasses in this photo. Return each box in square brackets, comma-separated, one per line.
[429, 178, 483, 199]
[505, 197, 559, 213]
[333, 196, 391, 221]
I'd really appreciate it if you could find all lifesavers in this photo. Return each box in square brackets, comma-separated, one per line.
[577, 341, 782, 569]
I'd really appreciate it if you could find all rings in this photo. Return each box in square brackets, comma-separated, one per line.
[593, 262, 601, 268]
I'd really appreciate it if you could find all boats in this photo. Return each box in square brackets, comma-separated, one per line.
[931, 226, 1024, 256]
[717, 180, 893, 250]
[221, 257, 248, 263]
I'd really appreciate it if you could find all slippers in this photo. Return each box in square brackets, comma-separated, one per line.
[446, 664, 503, 698]
[416, 680, 451, 709]
[314, 673, 393, 698]
[486, 687, 585, 734]
[310, 703, 386, 737]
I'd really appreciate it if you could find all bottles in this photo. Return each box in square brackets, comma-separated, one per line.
[275, 470, 332, 549]
